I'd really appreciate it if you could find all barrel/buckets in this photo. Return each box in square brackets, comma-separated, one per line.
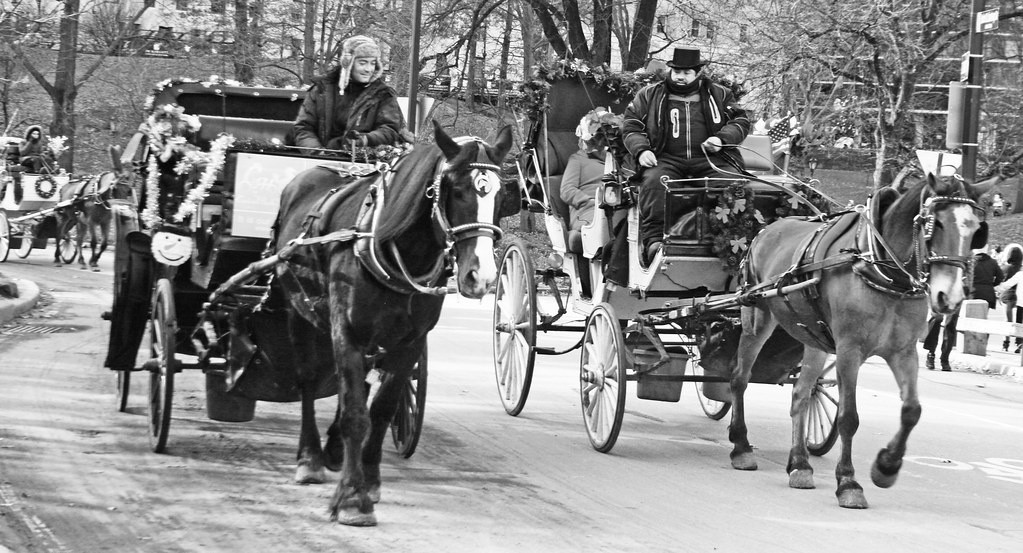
[631, 323, 689, 403]
[702, 370, 733, 403]
[203, 369, 256, 423]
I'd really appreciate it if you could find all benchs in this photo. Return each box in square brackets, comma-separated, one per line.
[189, 117, 298, 218]
[533, 133, 789, 237]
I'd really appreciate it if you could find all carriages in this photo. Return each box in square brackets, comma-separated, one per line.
[0, 133, 123, 273]
[489, 58, 1000, 507]
[104, 75, 514, 525]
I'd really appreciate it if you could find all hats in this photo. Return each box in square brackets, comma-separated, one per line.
[665, 47, 709, 69]
[337, 35, 383, 95]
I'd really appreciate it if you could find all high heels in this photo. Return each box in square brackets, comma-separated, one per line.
[1001, 340, 1010, 350]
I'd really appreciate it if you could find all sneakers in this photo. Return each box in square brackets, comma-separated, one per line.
[940, 356, 951, 371]
[1014, 344, 1022, 353]
[925, 353, 935, 369]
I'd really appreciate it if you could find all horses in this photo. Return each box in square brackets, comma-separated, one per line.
[273, 120, 514, 524]
[727, 170, 1001, 512]
[54, 171, 129, 272]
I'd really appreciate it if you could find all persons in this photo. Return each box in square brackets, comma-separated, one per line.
[968, 236, 1023, 355]
[19, 124, 47, 175]
[564, 46, 756, 293]
[291, 33, 407, 159]
